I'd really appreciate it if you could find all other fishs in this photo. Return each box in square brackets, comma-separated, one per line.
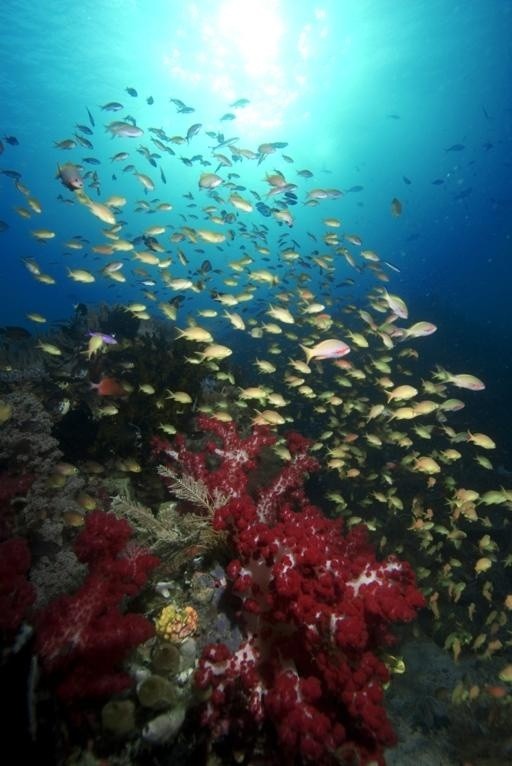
[0, 84, 512, 743]
